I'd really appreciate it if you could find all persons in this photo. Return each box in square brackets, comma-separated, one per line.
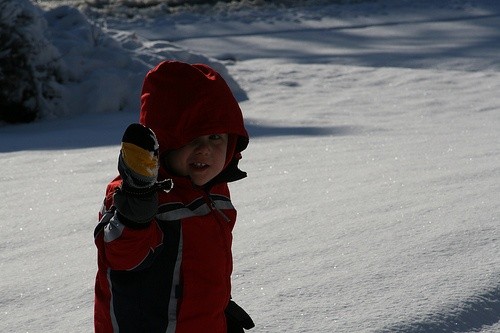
[92, 59, 260, 329]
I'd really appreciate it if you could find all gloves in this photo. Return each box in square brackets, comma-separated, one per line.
[229, 301, 254, 333]
[116, 123, 175, 195]
